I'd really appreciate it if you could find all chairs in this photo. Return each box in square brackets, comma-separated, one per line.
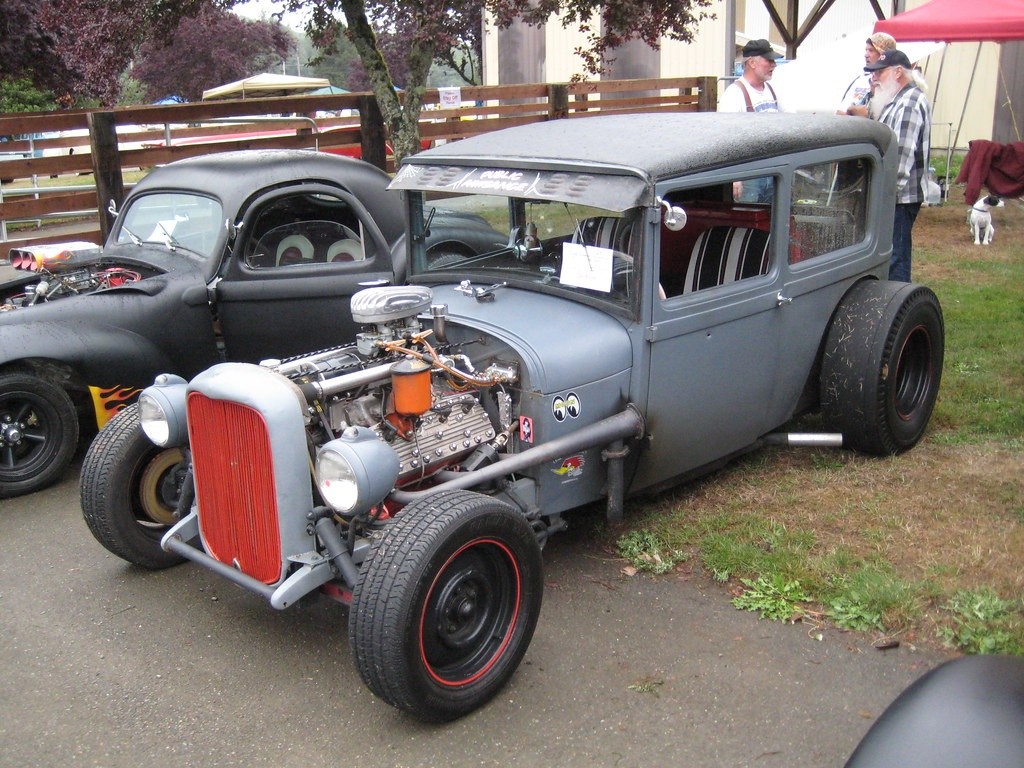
[571, 216, 636, 278]
[676, 225, 772, 296]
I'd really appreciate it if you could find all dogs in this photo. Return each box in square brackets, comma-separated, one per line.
[970, 194, 1007, 245]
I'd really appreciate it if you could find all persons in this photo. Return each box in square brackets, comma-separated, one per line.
[720, 38, 784, 201]
[838, 31, 896, 187]
[865, 50, 932, 283]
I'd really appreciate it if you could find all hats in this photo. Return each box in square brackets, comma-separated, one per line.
[863, 49, 911, 73]
[743, 39, 785, 61]
[870, 32, 897, 56]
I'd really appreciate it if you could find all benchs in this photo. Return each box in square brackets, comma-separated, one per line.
[262, 217, 365, 268]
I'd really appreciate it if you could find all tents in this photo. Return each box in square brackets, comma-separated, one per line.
[872, 0, 1024, 44]
[152, 96, 188, 104]
[311, 85, 351, 94]
[201, 73, 335, 99]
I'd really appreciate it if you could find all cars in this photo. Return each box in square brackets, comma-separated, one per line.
[78, 114, 946, 710]
[0, 149, 515, 499]
